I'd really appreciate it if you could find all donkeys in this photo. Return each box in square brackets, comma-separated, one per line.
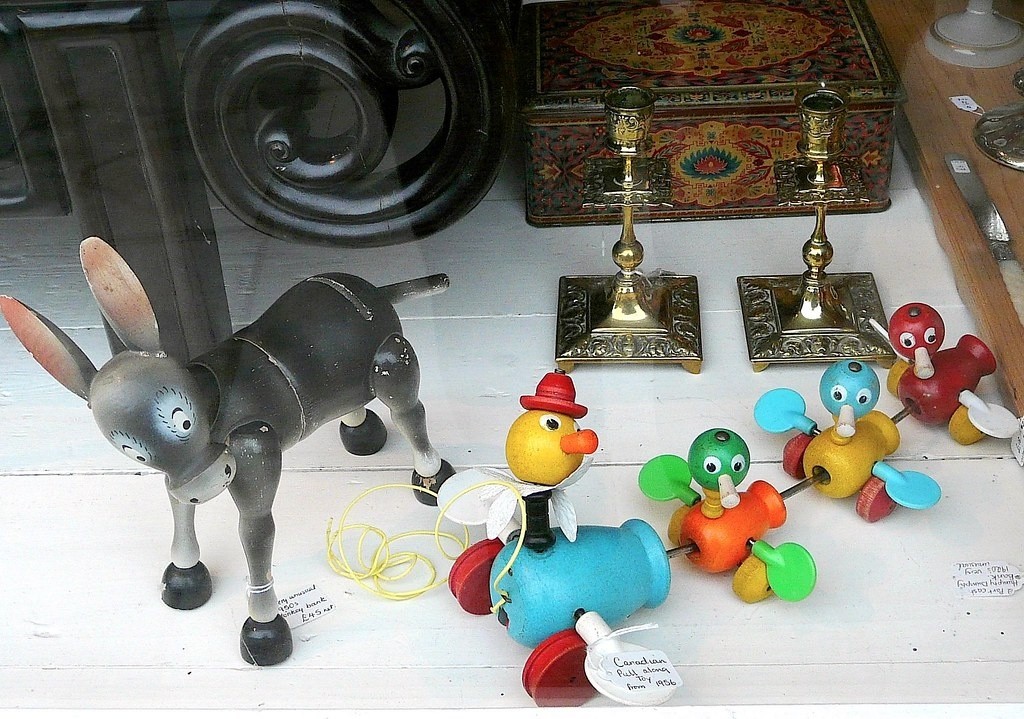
[1, 236, 450, 666]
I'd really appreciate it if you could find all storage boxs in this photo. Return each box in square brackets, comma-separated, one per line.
[523, 0, 909, 228]
